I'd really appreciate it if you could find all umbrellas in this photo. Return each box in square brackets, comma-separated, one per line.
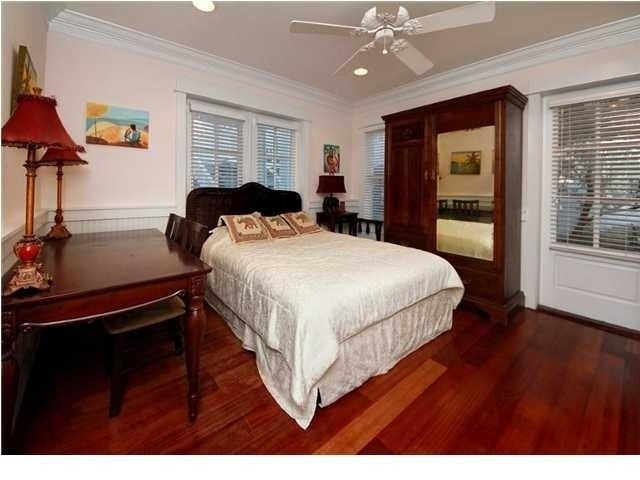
[88, 121, 112, 136]
[86, 103, 110, 136]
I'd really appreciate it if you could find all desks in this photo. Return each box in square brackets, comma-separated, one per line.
[2, 227, 211, 451]
[317, 212, 358, 236]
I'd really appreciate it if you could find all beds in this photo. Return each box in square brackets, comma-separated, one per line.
[436, 217, 494, 261]
[186, 182, 452, 400]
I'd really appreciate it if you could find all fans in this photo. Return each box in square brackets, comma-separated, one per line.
[290, 2, 496, 80]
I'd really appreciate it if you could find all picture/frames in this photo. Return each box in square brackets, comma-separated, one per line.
[11, 45, 39, 113]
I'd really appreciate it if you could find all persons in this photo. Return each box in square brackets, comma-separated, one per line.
[123, 123, 141, 144]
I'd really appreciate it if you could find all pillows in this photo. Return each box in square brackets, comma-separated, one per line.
[220, 211, 321, 242]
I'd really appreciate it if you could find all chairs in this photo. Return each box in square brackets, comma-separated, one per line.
[90, 213, 211, 418]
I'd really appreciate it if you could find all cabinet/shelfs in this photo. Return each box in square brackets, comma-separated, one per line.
[381, 84, 529, 327]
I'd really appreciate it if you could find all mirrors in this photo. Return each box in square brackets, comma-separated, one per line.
[435, 125, 497, 263]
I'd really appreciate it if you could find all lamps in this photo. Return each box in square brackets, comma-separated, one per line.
[0, 94, 88, 296]
[316, 176, 346, 212]
